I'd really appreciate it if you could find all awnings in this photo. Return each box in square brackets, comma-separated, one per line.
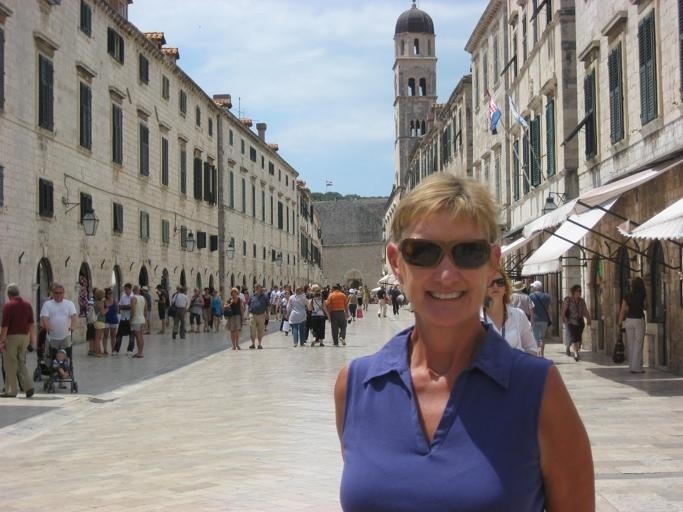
[377, 273, 400, 293]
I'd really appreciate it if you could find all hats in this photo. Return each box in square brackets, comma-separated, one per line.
[512, 281, 526, 292]
[530, 281, 542, 288]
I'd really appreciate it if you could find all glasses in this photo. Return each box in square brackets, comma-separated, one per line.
[401, 239, 491, 267]
[488, 279, 505, 286]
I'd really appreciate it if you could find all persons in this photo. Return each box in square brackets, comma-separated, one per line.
[618, 277, 648, 374]
[334, 173, 594, 510]
[561, 285, 591, 360]
[479, 269, 552, 358]
[272, 283, 348, 347]
[78, 274, 151, 358]
[377, 284, 402, 317]
[347, 286, 370, 321]
[0, 282, 78, 398]
[155, 285, 222, 339]
[223, 284, 270, 350]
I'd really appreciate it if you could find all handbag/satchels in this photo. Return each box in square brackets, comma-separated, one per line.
[614, 343, 624, 362]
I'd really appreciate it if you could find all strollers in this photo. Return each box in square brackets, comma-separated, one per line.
[32, 328, 49, 383]
[43, 327, 79, 395]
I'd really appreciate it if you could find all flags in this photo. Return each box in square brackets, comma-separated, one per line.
[482, 95, 503, 135]
[509, 97, 531, 133]
[326, 177, 337, 187]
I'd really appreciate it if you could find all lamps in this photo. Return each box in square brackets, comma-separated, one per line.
[65, 202, 100, 238]
[226, 240, 236, 261]
[176, 227, 196, 253]
[541, 191, 566, 217]
[268, 251, 283, 267]
